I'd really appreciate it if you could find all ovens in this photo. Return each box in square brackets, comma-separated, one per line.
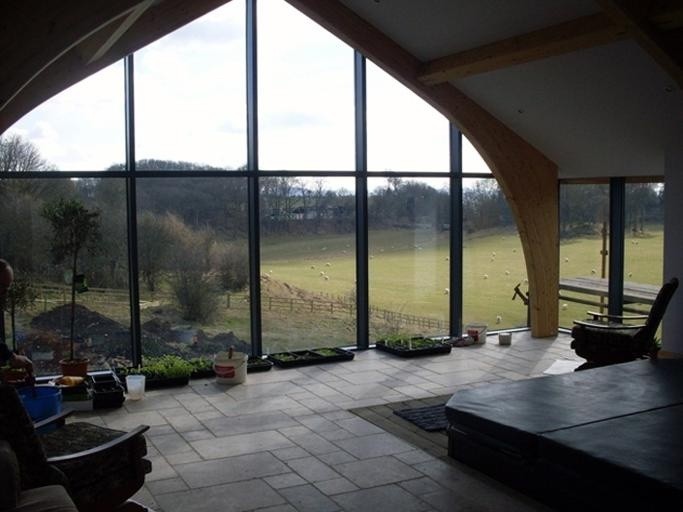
[445, 359, 682, 511]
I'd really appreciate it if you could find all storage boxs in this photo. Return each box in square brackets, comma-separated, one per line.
[559, 276, 664, 316]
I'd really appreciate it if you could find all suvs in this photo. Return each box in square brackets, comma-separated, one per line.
[212, 350, 248, 387]
[126, 375, 144, 401]
[465, 324, 487, 344]
[16, 386, 63, 433]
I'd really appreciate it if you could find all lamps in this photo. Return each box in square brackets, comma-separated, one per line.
[571, 277, 679, 371]
[0, 383, 152, 512]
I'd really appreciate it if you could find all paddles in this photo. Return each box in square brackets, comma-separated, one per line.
[393, 403, 450, 432]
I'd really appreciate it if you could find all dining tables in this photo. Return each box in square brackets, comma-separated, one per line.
[374, 332, 452, 358]
[55, 347, 355, 410]
